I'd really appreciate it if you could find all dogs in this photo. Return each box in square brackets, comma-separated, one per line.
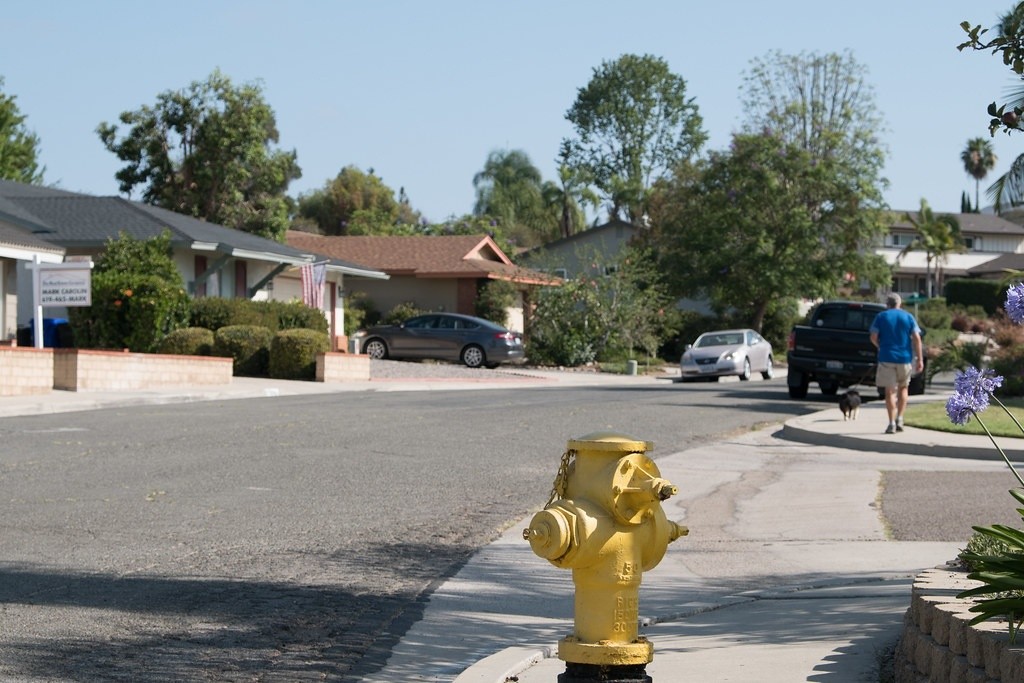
[840, 390, 861, 420]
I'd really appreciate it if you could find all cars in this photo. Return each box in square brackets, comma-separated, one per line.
[680, 328, 775, 382]
[358, 311, 524, 370]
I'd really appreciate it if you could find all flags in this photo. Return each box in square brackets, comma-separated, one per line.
[302, 261, 326, 311]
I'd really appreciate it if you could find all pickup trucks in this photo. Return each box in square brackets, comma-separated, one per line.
[786, 300, 927, 400]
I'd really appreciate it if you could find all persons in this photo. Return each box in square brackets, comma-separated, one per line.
[870, 293, 923, 434]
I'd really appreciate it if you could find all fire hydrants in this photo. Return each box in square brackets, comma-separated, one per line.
[522, 425, 690, 683]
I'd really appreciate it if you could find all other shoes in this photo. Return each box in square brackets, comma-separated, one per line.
[885, 424, 896, 434]
[895, 420, 903, 431]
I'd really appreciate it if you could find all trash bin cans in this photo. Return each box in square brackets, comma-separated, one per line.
[17, 325, 31, 346]
[29, 317, 70, 347]
